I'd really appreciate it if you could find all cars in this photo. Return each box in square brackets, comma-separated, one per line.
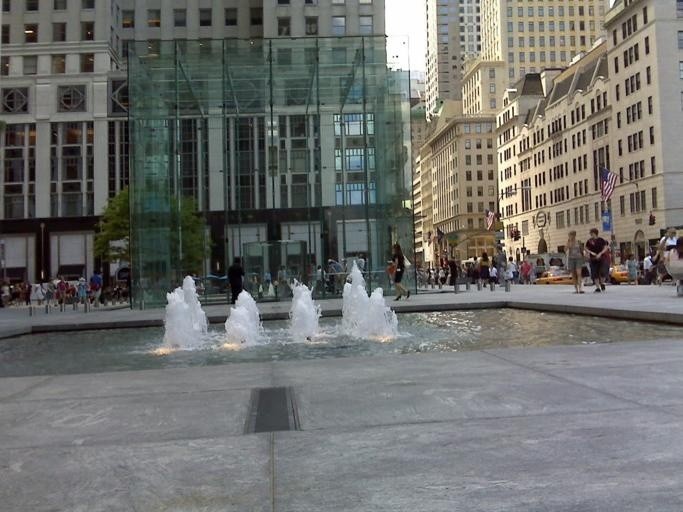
[462, 254, 670, 286]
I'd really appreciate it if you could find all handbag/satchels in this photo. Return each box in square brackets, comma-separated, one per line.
[582, 266, 589, 277]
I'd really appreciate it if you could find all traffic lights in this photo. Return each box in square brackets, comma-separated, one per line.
[648, 216, 656, 226]
[514, 229, 521, 241]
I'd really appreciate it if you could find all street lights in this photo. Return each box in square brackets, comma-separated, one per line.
[253, 241, 273, 286]
[494, 178, 533, 254]
[278, 238, 295, 282]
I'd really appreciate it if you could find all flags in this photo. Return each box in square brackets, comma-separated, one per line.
[483, 208, 494, 230]
[600, 167, 618, 203]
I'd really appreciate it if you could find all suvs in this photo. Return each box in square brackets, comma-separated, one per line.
[112, 261, 157, 291]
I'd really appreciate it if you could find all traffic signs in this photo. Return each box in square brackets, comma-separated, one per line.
[492, 221, 503, 230]
[495, 243, 504, 247]
[494, 232, 504, 240]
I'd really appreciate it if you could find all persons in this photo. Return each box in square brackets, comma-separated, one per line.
[220, 249, 367, 305]
[421, 228, 682, 296]
[1, 268, 104, 311]
[387, 242, 411, 301]
